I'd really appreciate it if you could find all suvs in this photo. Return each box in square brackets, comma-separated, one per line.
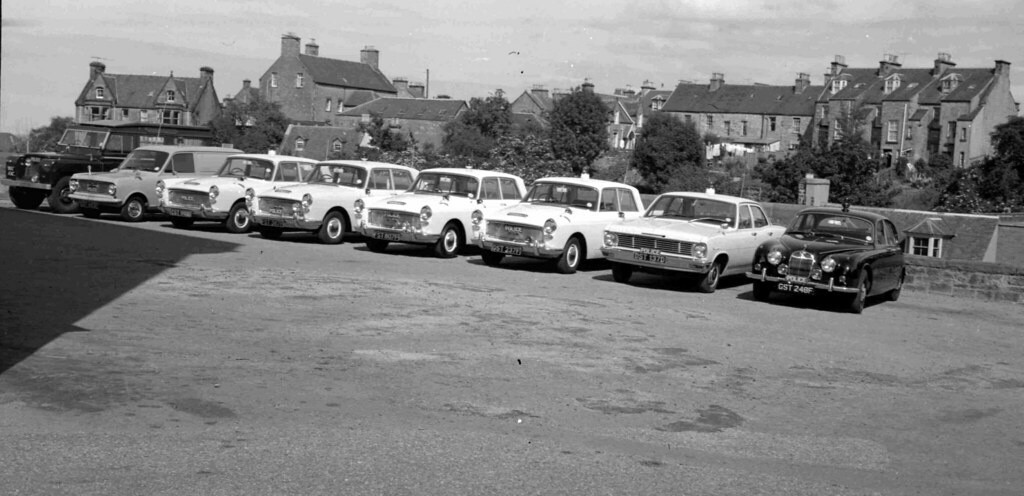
[0, 122, 216, 215]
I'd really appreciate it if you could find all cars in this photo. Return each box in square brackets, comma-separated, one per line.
[481, 174, 662, 275]
[68, 147, 525, 276]
[746, 203, 906, 313]
[600, 190, 788, 293]
